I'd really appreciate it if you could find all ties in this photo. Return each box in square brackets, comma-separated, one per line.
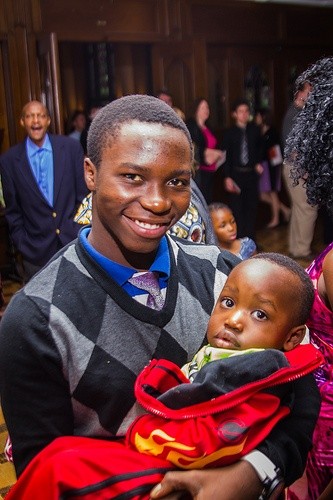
[39, 150, 49, 197]
[128, 271, 165, 311]
[241, 129, 248, 165]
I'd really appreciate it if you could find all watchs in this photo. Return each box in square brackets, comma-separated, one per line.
[240, 449, 285, 500]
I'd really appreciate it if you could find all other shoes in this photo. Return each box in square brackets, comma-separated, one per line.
[288, 253, 319, 261]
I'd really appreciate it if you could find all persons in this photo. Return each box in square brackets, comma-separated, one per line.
[0, 83, 319, 283]
[3, 253, 324, 500]
[0, 94, 321, 500]
[286, 58, 333, 500]
